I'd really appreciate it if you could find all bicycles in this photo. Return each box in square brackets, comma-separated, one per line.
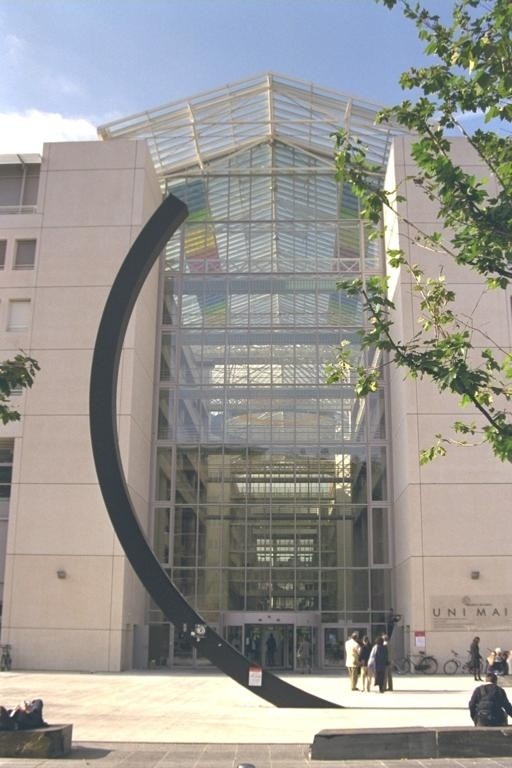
[1, 645, 11, 670]
[392, 650, 483, 675]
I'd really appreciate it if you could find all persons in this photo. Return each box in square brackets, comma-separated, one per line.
[467, 673, 512, 726]
[381, 633, 393, 692]
[366, 637, 389, 693]
[470, 636, 483, 681]
[486, 648, 510, 677]
[230, 631, 312, 675]
[344, 633, 362, 690]
[358, 635, 372, 692]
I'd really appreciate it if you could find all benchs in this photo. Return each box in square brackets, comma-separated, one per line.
[311, 726, 512, 759]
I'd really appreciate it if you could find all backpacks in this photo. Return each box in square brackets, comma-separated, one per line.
[474, 684, 506, 725]
